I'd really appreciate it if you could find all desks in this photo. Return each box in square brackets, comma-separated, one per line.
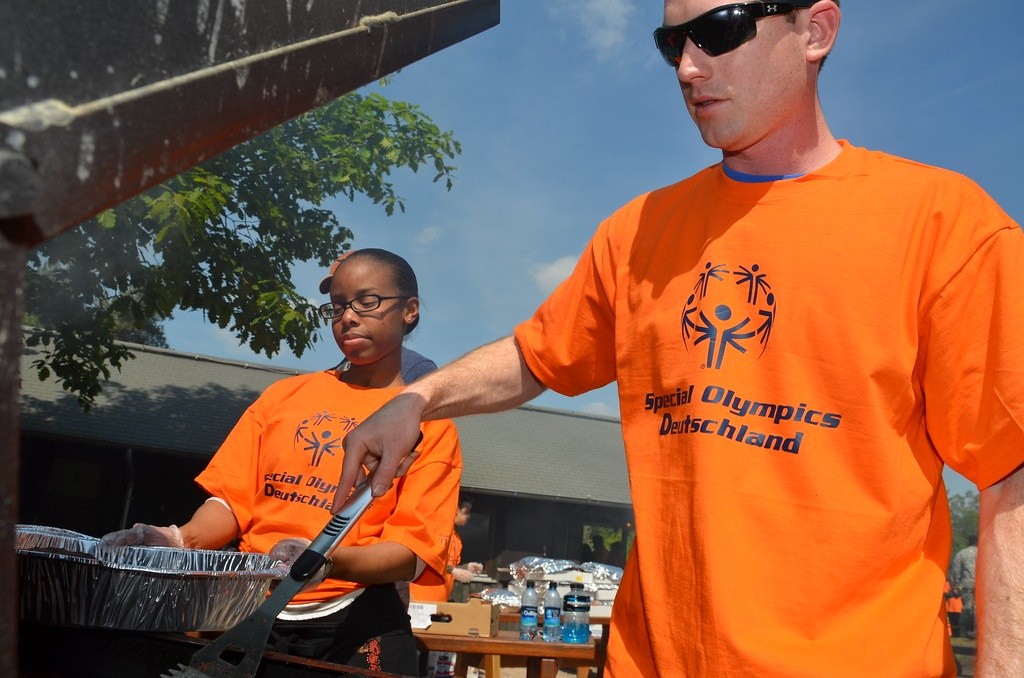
[454, 606, 612, 678]
[413, 630, 596, 678]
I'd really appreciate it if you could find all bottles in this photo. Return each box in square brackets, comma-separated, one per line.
[521, 580, 537, 640]
[561, 584, 591, 644]
[543, 582, 561, 642]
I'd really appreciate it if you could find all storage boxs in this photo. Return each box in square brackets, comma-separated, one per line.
[407, 597, 500, 638]
[509, 570, 617, 601]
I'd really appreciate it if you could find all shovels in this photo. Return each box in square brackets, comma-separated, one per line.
[159, 424, 424, 678]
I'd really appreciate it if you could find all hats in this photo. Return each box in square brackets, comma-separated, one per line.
[318, 250, 357, 296]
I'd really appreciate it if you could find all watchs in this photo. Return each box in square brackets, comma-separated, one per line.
[322, 556, 333, 583]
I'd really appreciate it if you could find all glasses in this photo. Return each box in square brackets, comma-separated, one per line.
[319, 294, 411, 320]
[652, 0, 814, 67]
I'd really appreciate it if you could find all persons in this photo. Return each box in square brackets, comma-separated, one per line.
[331, 0, 1024, 678]
[102, 249, 627, 678]
